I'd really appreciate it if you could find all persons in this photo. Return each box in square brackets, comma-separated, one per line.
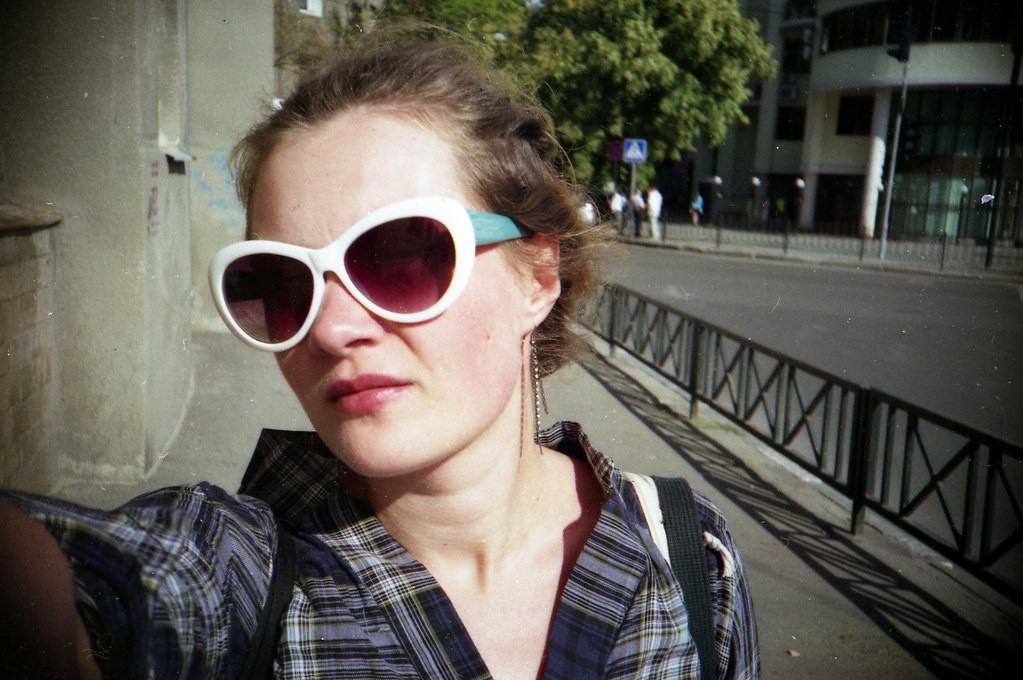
[690, 191, 703, 225]
[609, 188, 624, 236]
[632, 188, 645, 237]
[646, 184, 663, 239]
[0, 20, 758, 680]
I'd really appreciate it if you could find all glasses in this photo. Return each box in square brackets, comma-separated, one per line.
[206, 197, 533, 354]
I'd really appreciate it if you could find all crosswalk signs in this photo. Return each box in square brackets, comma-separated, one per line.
[623, 138, 647, 162]
